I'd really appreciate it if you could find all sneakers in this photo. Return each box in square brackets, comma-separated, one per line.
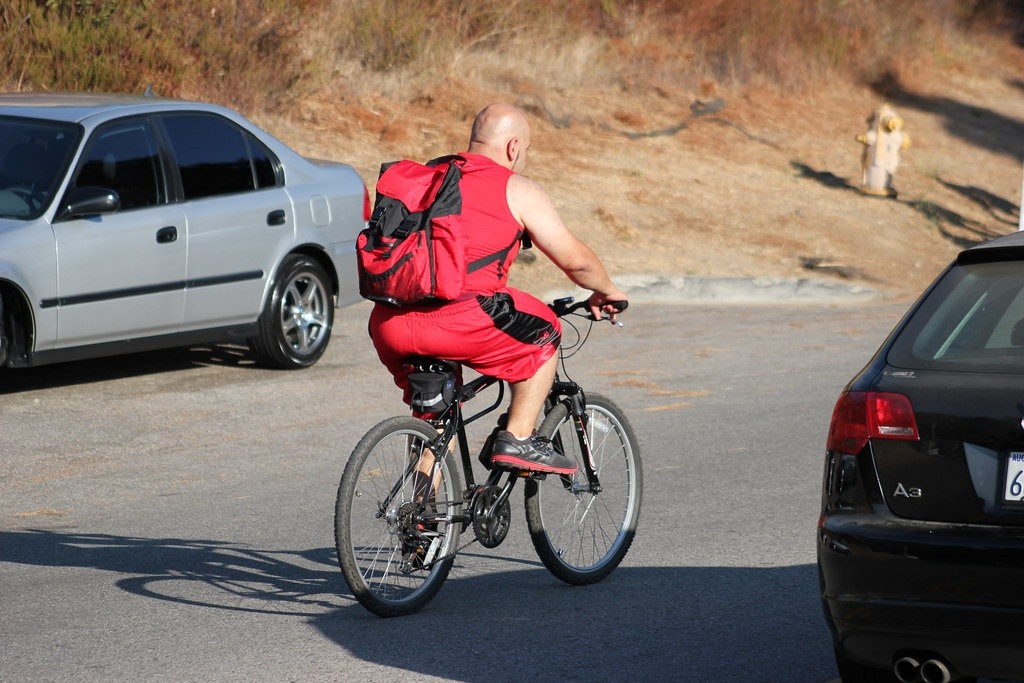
[490, 427, 578, 474]
[402, 521, 438, 568]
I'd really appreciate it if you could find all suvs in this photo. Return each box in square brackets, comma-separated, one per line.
[812, 228, 1024, 683]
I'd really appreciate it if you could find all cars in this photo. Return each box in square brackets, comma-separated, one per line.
[0, 88, 375, 389]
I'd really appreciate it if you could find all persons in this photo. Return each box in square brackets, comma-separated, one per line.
[368, 102, 628, 570]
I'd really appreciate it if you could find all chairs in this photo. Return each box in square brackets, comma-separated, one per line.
[4, 141, 45, 183]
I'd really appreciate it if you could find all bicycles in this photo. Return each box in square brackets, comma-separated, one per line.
[331, 296, 646, 620]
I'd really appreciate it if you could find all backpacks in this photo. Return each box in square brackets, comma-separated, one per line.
[355, 155, 533, 306]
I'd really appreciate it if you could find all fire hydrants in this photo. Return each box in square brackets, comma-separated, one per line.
[853, 105, 912, 199]
